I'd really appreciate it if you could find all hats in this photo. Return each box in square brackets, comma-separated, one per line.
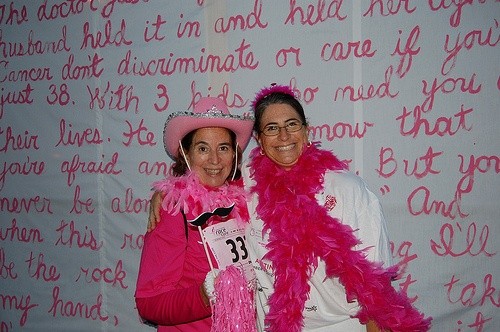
[162, 97, 256, 163]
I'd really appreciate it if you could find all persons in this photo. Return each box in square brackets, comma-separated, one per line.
[146, 85, 391, 332]
[134, 97, 256, 332]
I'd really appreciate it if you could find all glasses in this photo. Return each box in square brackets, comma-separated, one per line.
[259, 120, 307, 136]
